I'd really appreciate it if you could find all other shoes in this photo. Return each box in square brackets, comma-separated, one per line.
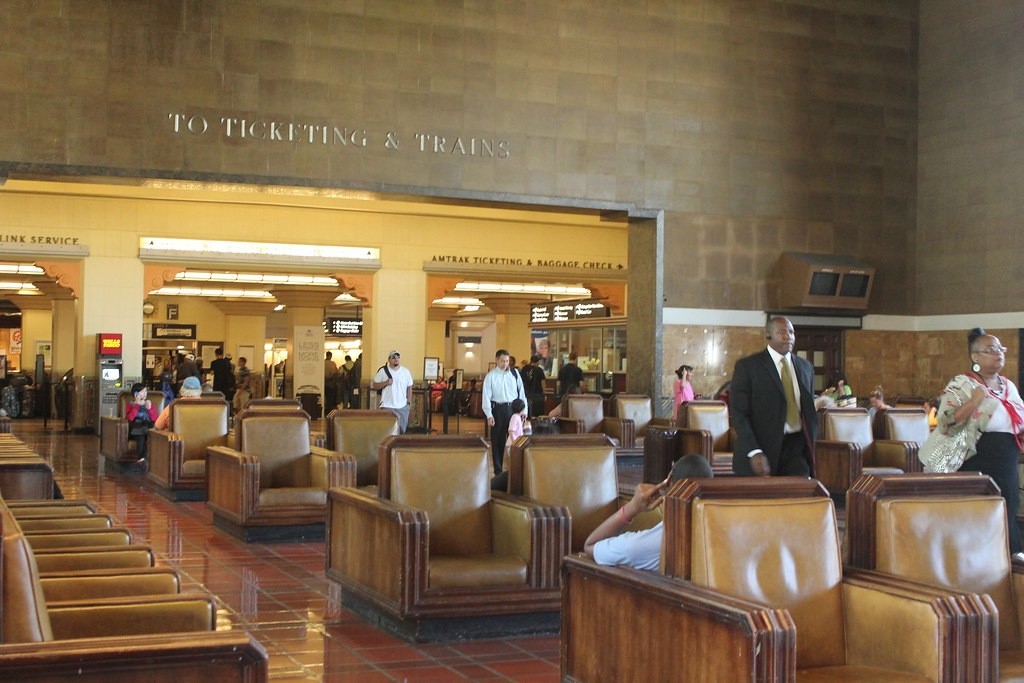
[1012, 552, 1023, 565]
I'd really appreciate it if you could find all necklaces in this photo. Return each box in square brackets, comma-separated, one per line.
[976, 372, 1003, 395]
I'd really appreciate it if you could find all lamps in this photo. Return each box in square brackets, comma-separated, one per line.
[175, 273, 340, 286]
[0, 262, 45, 275]
[454, 282, 592, 298]
[0, 280, 39, 291]
[149, 286, 275, 298]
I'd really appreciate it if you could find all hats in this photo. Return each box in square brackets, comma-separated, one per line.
[389, 350, 401, 358]
[183, 377, 200, 389]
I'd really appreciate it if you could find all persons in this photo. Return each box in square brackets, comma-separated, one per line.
[674, 366, 694, 416]
[325, 352, 361, 409]
[154, 376, 202, 430]
[235, 357, 248, 390]
[153, 353, 205, 386]
[822, 371, 852, 402]
[431, 376, 446, 413]
[583, 454, 714, 573]
[937, 327, 1024, 562]
[557, 352, 585, 397]
[539, 340, 552, 372]
[730, 316, 819, 479]
[126, 383, 156, 462]
[266, 359, 286, 397]
[868, 385, 893, 417]
[482, 350, 546, 475]
[210, 349, 234, 399]
[373, 350, 413, 434]
[449, 369, 479, 416]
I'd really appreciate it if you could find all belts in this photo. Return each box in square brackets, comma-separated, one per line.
[496, 402, 512, 406]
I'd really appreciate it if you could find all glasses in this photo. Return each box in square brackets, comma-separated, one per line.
[975, 347, 1006, 355]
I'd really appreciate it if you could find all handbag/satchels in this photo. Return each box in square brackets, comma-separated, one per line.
[918, 376, 978, 473]
[377, 364, 392, 394]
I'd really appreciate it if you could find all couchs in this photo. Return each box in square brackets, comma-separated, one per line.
[0, 370, 1024, 683]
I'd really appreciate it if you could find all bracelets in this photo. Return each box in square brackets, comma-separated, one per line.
[621, 506, 633, 524]
[408, 402, 411, 405]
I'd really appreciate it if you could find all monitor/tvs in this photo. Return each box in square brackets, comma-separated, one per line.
[102, 368, 120, 381]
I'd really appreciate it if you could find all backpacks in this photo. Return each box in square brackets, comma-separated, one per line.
[342, 364, 355, 391]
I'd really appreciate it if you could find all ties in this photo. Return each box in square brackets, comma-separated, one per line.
[781, 356, 799, 431]
